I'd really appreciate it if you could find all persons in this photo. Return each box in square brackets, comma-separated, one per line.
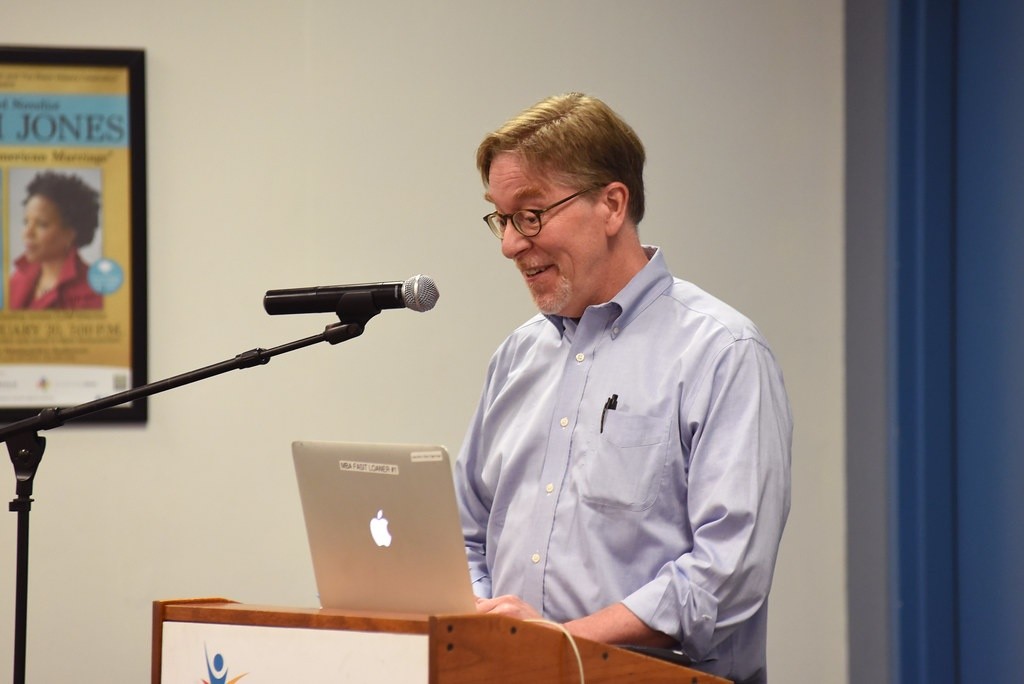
[452, 91, 794, 684]
[8, 172, 103, 309]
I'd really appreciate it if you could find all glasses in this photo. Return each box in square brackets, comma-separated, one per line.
[484, 182, 609, 239]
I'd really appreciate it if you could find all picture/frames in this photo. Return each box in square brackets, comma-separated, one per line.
[0, 45, 147, 425]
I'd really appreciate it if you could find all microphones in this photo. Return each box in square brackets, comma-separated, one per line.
[263, 273, 440, 315]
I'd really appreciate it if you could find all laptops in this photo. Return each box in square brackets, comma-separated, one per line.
[288, 439, 478, 615]
